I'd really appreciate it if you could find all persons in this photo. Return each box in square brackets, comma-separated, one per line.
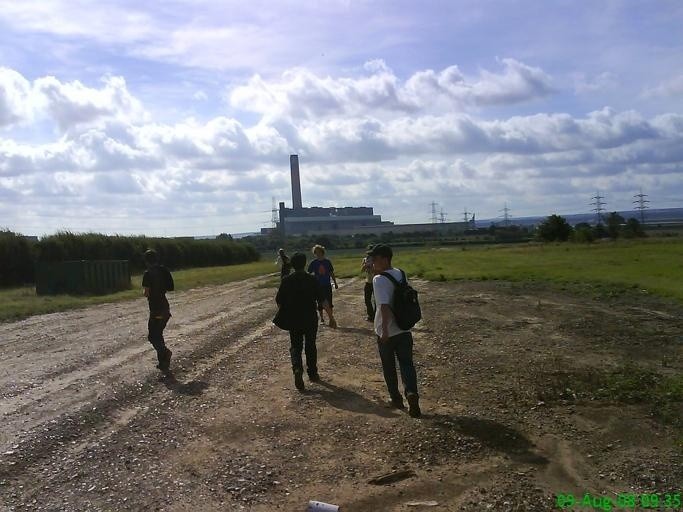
[307, 243, 339, 329]
[371, 243, 422, 419]
[276, 248, 292, 280]
[273, 253, 325, 391]
[140, 248, 175, 371]
[360, 244, 376, 324]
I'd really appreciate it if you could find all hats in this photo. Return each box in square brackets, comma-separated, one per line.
[364, 243, 392, 257]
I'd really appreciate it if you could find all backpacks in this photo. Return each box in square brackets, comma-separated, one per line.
[371, 268, 422, 331]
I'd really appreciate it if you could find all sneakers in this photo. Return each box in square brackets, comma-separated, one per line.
[308, 374, 318, 380]
[406, 392, 422, 418]
[364, 314, 371, 321]
[293, 368, 304, 390]
[388, 398, 403, 408]
